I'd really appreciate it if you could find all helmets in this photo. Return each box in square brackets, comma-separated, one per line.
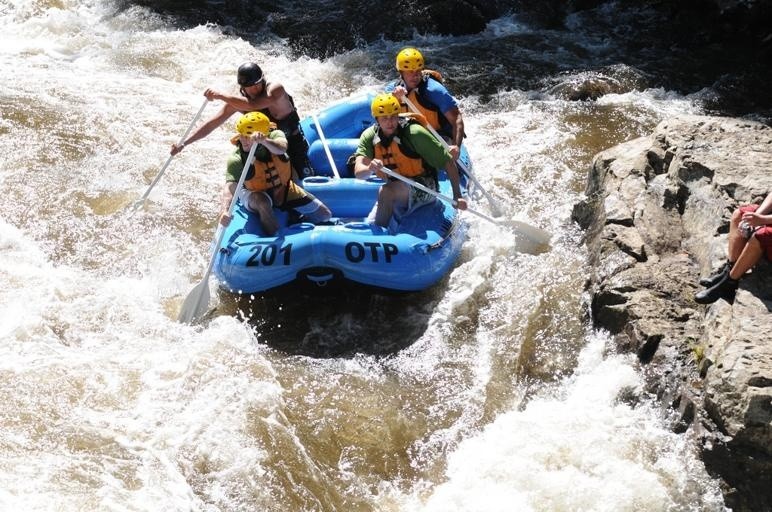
[371, 95, 401, 116]
[238, 62, 263, 86]
[396, 49, 424, 71]
[236, 112, 270, 137]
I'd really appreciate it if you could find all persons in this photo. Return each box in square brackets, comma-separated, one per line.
[385, 47, 464, 163]
[692, 192, 771, 304]
[171, 60, 314, 180]
[218, 111, 340, 236]
[354, 93, 467, 228]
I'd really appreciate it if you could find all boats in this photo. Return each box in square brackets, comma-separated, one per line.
[213, 91, 471, 295]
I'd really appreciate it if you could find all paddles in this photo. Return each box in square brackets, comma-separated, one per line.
[402, 95, 506, 218]
[178, 141, 258, 325]
[381, 168, 552, 246]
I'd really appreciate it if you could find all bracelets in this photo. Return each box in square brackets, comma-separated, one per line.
[182, 143, 186, 147]
